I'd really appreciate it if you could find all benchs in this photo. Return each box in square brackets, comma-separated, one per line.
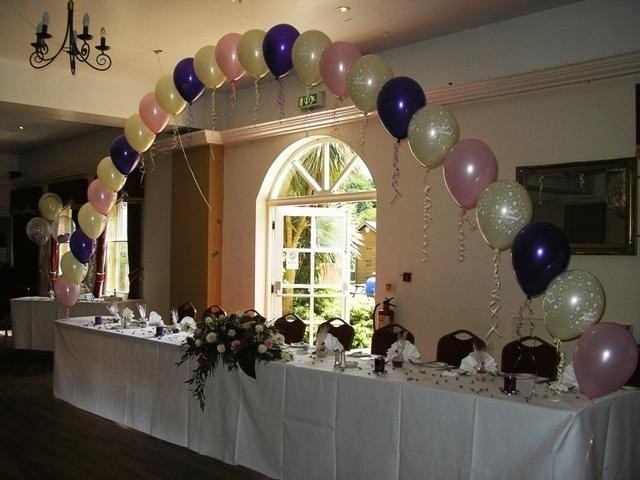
[350, 285, 375, 298]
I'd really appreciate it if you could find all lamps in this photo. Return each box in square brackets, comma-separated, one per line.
[29, 0, 112, 75]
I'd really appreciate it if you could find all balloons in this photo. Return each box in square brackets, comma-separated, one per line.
[319, 41, 360, 96]
[54, 178, 117, 309]
[39, 193, 62, 220]
[574, 323, 638, 401]
[442, 139, 498, 209]
[154, 29, 269, 118]
[543, 270, 605, 340]
[51, 216, 76, 244]
[511, 222, 570, 298]
[376, 76, 425, 139]
[97, 91, 170, 196]
[291, 30, 331, 85]
[27, 217, 50, 245]
[348, 54, 392, 112]
[477, 179, 532, 250]
[408, 104, 458, 169]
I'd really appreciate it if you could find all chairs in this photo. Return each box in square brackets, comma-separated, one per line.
[168, 301, 640, 388]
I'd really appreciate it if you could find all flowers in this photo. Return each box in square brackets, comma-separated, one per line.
[175, 316, 295, 414]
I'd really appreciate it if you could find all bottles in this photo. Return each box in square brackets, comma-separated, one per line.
[334, 349, 346, 368]
[121, 315, 127, 328]
[113, 288, 116, 296]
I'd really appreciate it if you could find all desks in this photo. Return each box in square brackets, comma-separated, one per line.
[9, 296, 147, 352]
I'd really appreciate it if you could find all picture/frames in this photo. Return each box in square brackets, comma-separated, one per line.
[516, 157, 637, 255]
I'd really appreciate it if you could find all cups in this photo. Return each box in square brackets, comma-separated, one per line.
[503, 377, 516, 390]
[95, 317, 101, 325]
[374, 358, 385, 373]
[156, 326, 162, 336]
[26, 288, 31, 296]
[393, 359, 403, 368]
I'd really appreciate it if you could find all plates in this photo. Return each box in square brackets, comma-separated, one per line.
[426, 362, 447, 367]
[291, 343, 309, 347]
[515, 373, 535, 379]
[348, 353, 371, 357]
